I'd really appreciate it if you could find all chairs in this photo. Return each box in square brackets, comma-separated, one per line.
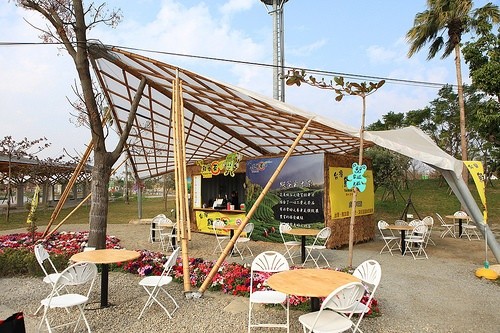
[35, 245, 97, 333]
[138, 211, 483, 333]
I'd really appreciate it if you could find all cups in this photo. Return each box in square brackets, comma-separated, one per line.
[230, 205, 235, 210]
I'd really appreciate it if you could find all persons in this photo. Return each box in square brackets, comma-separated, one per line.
[227, 191, 239, 209]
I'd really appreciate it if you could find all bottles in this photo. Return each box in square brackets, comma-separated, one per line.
[227, 201, 231, 210]
[241, 202, 245, 210]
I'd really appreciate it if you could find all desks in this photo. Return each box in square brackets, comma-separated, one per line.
[445, 216, 468, 238]
[218, 226, 240, 255]
[69, 248, 141, 307]
[267, 270, 362, 312]
[386, 226, 416, 255]
[161, 225, 179, 251]
[285, 229, 320, 263]
